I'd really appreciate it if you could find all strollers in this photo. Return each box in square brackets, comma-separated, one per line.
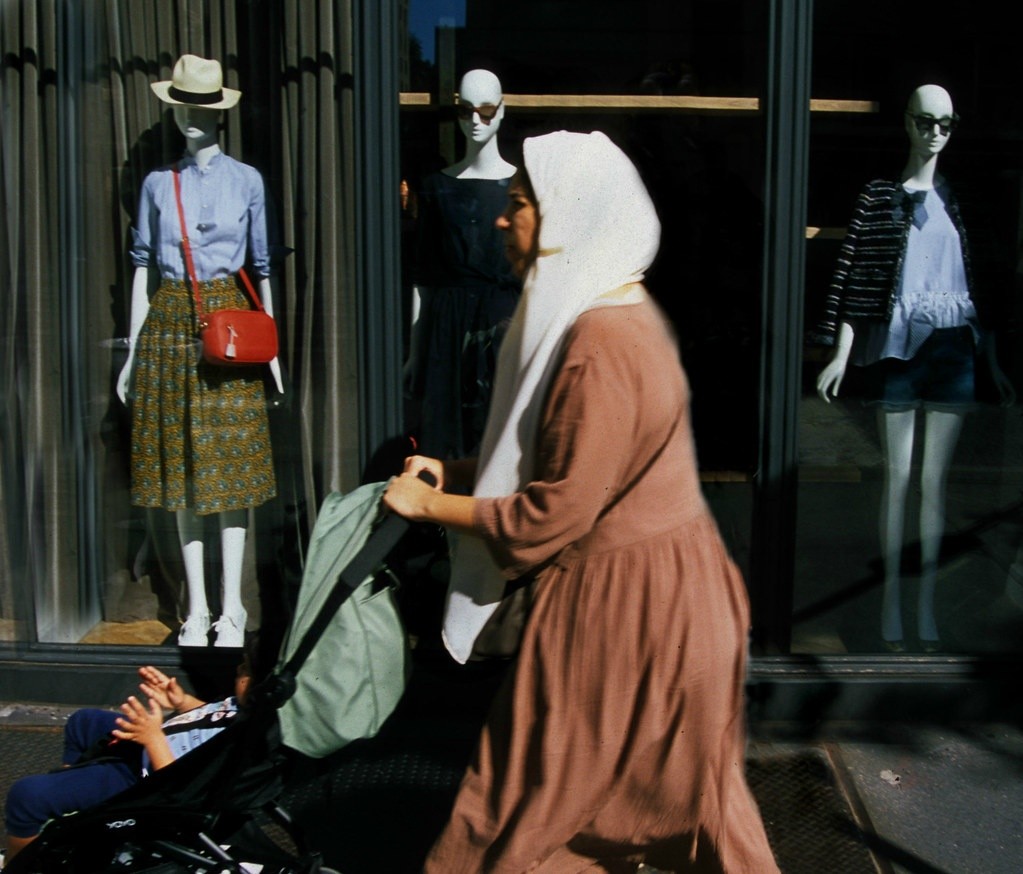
[0, 468, 496, 874]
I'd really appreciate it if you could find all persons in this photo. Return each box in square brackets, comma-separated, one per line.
[117, 54, 283, 646]
[401, 69, 528, 464]
[815, 84, 1007, 654]
[8, 635, 267, 855]
[380, 130, 783, 874]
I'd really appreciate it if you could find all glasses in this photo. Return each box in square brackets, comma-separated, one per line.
[909, 112, 954, 130]
[453, 98, 502, 119]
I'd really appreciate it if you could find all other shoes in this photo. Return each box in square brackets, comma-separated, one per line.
[882, 637, 906, 654]
[924, 639, 944, 653]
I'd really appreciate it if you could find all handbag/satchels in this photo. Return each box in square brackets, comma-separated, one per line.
[468, 584, 528, 667]
[200, 309, 277, 364]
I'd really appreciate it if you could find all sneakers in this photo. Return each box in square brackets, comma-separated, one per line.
[178, 612, 213, 646]
[211, 608, 247, 647]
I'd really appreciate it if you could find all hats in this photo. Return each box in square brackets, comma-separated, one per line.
[149, 54, 242, 109]
